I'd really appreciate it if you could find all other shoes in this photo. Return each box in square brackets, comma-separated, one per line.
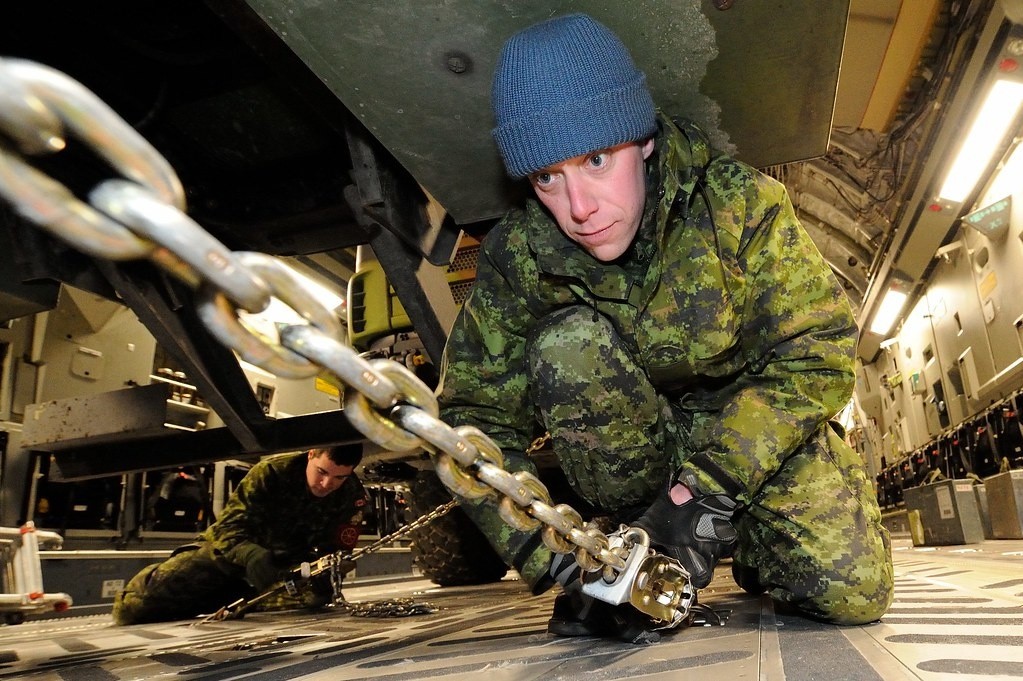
[547, 591, 589, 634]
[732, 558, 772, 593]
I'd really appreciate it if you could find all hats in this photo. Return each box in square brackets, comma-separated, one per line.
[490, 14, 657, 178]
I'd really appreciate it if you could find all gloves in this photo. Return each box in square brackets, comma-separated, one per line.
[260, 551, 302, 584]
[547, 550, 660, 645]
[309, 574, 334, 601]
[624, 490, 738, 589]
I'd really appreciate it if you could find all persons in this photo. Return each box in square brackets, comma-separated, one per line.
[112, 441, 371, 624]
[144, 465, 206, 530]
[429, 10, 895, 640]
